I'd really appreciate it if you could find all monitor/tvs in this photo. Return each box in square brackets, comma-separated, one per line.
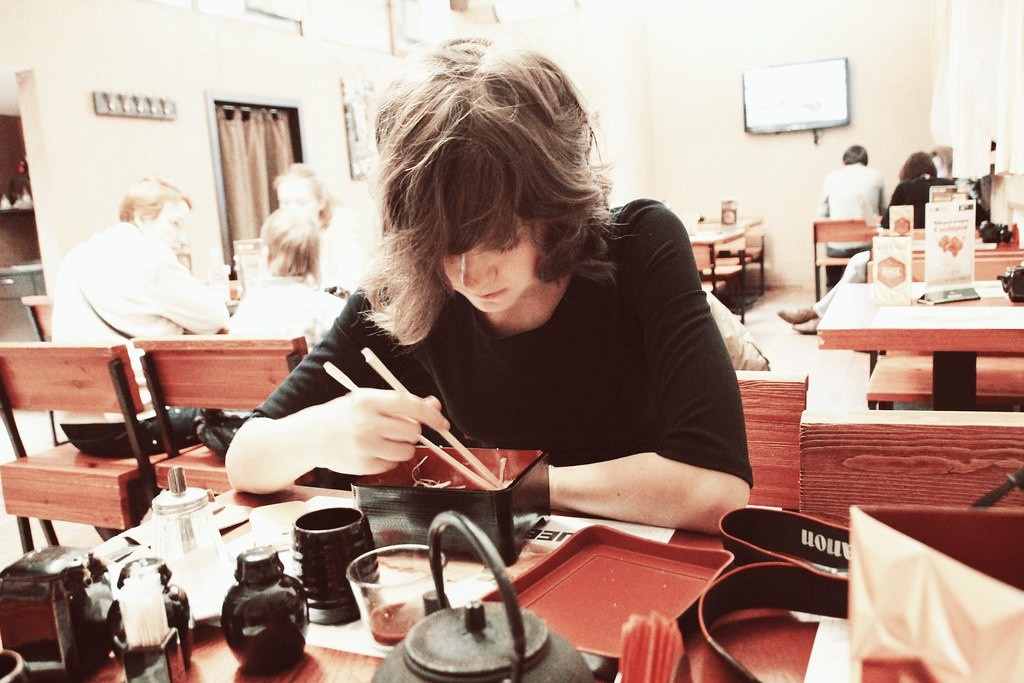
[741, 56, 849, 135]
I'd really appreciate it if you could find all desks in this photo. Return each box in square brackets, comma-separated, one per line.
[818, 281, 1024, 412]
[90, 487, 819, 683]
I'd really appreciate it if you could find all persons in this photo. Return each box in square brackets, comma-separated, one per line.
[51, 176, 230, 458]
[230, 201, 348, 354]
[818, 145, 888, 288]
[930, 146, 953, 177]
[699, 286, 771, 372]
[777, 151, 989, 335]
[278, 160, 376, 297]
[224, 37, 754, 535]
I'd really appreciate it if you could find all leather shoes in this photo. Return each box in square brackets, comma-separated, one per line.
[777, 306, 817, 325]
[794, 317, 820, 335]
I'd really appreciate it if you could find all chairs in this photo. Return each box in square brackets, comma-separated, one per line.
[0, 218, 1024, 555]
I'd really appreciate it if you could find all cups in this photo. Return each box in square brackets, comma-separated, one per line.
[0, 649, 33, 683]
[292, 508, 379, 626]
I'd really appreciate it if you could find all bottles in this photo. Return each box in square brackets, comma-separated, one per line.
[220, 546, 313, 673]
[103, 559, 193, 667]
[151, 467, 239, 626]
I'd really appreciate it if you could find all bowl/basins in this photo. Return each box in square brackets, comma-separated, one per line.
[345, 542, 447, 650]
[350, 445, 551, 566]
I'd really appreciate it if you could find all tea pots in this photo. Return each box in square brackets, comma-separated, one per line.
[997, 264, 1024, 302]
[376, 509, 600, 683]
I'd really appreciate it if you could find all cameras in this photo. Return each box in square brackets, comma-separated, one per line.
[979, 221, 1012, 243]
[1002, 266, 1024, 302]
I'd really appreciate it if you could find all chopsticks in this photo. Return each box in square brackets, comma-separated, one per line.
[323, 347, 505, 490]
